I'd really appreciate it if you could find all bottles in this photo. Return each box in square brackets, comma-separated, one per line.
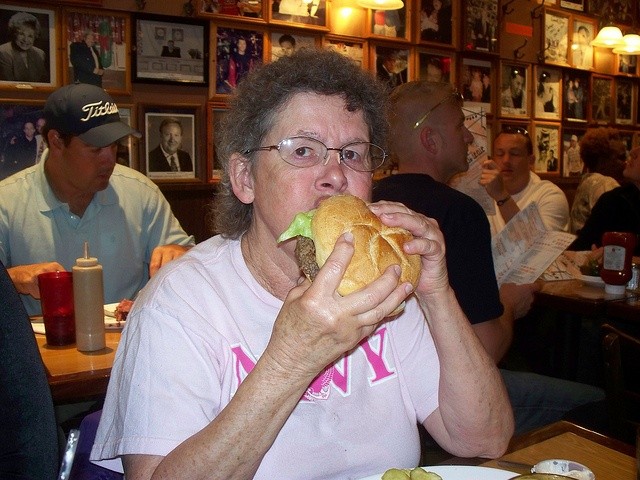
[600, 231, 635, 296]
[72, 242, 107, 356]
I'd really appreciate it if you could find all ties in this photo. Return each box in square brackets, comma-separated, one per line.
[171, 157, 178, 171]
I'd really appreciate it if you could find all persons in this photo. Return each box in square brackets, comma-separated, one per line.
[149, 117, 193, 172]
[11, 111, 35, 175]
[229, 35, 253, 92]
[421, 0, 450, 40]
[570, 126, 627, 234]
[89, 47, 515, 480]
[536, 71, 557, 113]
[0, 83, 197, 320]
[277, 35, 297, 59]
[476, 126, 570, 241]
[502, 67, 526, 109]
[378, 50, 398, 91]
[0, 11, 47, 82]
[474, 9, 492, 50]
[577, 25, 594, 68]
[0, 260, 58, 480]
[465, 70, 490, 102]
[371, 80, 612, 432]
[426, 58, 445, 82]
[566, 142, 640, 268]
[566, 134, 582, 177]
[161, 40, 180, 57]
[566, 78, 585, 119]
[70, 33, 104, 86]
[35, 118, 48, 161]
[546, 151, 557, 171]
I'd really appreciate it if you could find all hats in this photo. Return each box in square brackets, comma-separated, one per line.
[44, 82, 142, 148]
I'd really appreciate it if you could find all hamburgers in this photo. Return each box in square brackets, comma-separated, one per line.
[274, 191, 426, 319]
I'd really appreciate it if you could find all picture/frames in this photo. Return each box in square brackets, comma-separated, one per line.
[205, 103, 234, 188]
[116, 103, 136, 171]
[560, 68, 593, 126]
[130, 11, 210, 87]
[614, 23, 640, 79]
[559, 126, 590, 185]
[615, 76, 638, 130]
[320, 34, 367, 76]
[491, 120, 530, 139]
[412, 47, 459, 101]
[267, 0, 334, 32]
[590, 72, 615, 130]
[368, 39, 414, 95]
[458, 52, 497, 119]
[268, 24, 317, 77]
[533, 65, 563, 122]
[61, 4, 133, 97]
[133, 101, 204, 188]
[413, 1, 459, 51]
[208, 19, 269, 102]
[571, 13, 599, 72]
[368, 0, 413, 44]
[531, 121, 560, 180]
[460, 0, 503, 58]
[193, 1, 267, 23]
[497, 58, 534, 122]
[0, 100, 50, 182]
[0, 0, 61, 98]
[538, 6, 571, 69]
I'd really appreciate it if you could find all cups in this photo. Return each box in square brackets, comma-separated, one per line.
[37, 270, 76, 346]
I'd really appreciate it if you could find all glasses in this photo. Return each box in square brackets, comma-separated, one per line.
[413, 90, 464, 129]
[244, 136, 390, 173]
[502, 127, 529, 137]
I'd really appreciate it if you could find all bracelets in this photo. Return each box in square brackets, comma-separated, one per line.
[497, 194, 511, 208]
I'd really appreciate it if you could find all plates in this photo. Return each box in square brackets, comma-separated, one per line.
[101, 299, 136, 328]
[358, 464, 527, 480]
[578, 273, 603, 283]
[526, 457, 598, 479]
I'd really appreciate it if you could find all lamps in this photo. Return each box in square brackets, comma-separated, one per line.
[590, 24, 624, 48]
[613, 29, 640, 58]
